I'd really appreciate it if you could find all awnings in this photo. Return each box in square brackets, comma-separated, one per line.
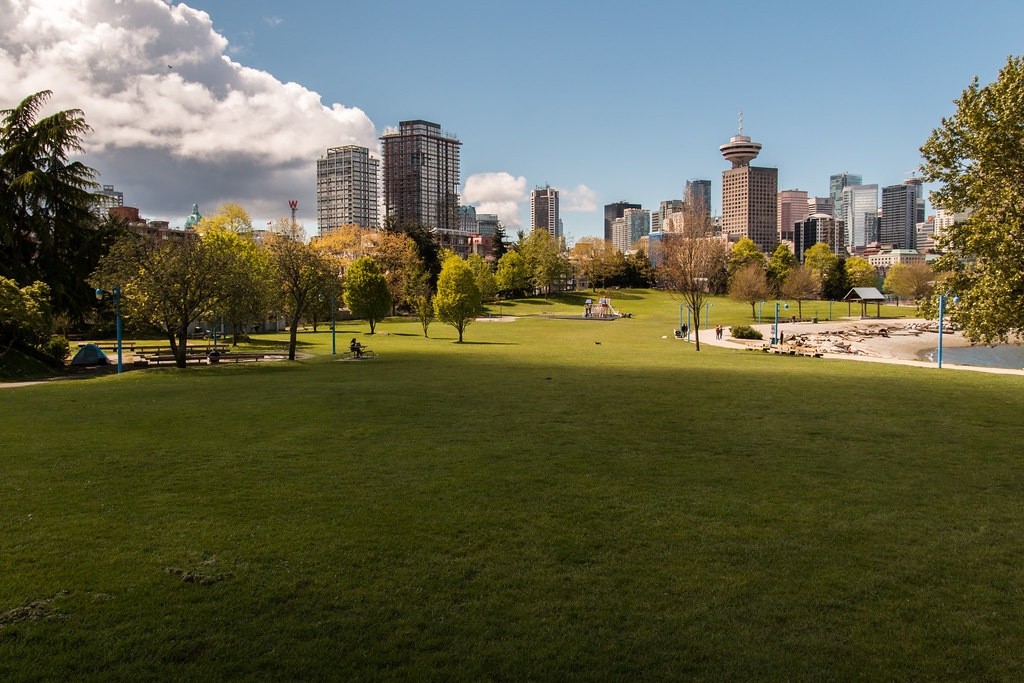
[844, 287, 886, 320]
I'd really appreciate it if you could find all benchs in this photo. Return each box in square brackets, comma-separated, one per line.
[358, 350, 376, 358]
[744, 341, 824, 358]
[345, 351, 357, 359]
[78, 344, 265, 366]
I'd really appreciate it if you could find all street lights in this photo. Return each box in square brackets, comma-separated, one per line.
[759, 299, 765, 325]
[774, 302, 789, 345]
[937, 288, 961, 370]
[95, 286, 124, 374]
[318, 293, 336, 355]
[705, 303, 714, 329]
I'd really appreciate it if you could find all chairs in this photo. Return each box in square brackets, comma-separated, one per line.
[208, 355, 222, 366]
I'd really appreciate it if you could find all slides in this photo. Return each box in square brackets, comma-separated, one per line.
[610, 304, 618, 315]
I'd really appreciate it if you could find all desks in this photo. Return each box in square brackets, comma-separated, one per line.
[136, 344, 230, 349]
[89, 342, 136, 346]
[348, 345, 368, 359]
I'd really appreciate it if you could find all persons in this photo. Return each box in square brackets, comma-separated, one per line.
[780, 330, 784, 345]
[681, 323, 686, 338]
[715, 325, 723, 340]
[792, 314, 796, 325]
[351, 338, 363, 359]
[208, 347, 221, 362]
